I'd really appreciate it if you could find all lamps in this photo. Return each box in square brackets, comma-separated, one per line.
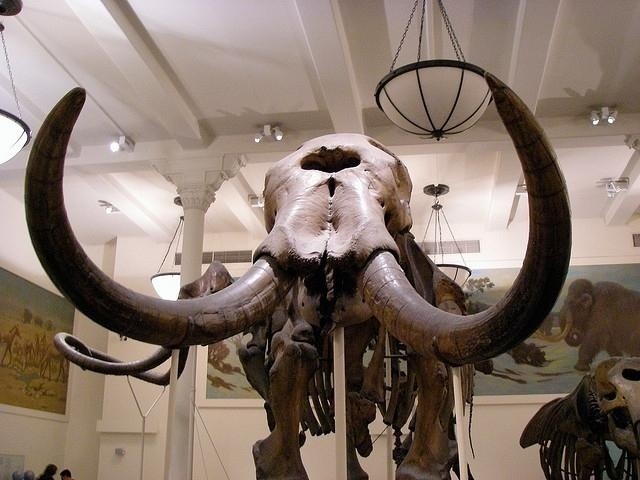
[151, 215, 185, 303]
[254, 124, 283, 143]
[420, 185, 472, 289]
[590, 106, 617, 126]
[374, 1, 494, 142]
[0, 0, 32, 165]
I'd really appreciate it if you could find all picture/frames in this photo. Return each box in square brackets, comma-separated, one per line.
[462, 257, 640, 405]
[0, 259, 80, 425]
[195, 268, 420, 406]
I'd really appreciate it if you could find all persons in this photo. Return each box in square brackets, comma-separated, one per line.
[60, 469, 74, 480]
[37, 464, 57, 480]
